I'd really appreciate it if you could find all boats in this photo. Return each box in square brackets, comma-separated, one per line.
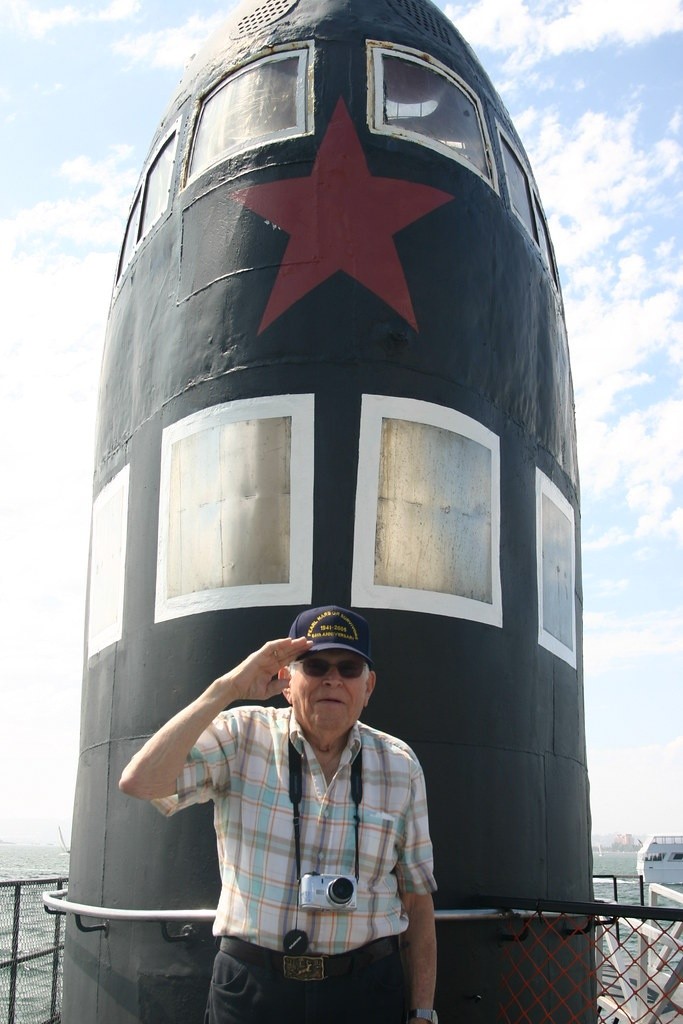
[637, 835, 683, 884]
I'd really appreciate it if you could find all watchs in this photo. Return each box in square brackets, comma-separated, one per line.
[406, 1008, 439, 1024]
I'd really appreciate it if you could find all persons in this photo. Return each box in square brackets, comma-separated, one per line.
[118, 605, 437, 1024]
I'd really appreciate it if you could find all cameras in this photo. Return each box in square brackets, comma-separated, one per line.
[299, 874, 357, 912]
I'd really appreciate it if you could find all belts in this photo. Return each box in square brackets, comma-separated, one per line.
[220, 936, 399, 981]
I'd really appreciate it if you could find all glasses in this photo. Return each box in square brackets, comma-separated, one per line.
[291, 659, 367, 679]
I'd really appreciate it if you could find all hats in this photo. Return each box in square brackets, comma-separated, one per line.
[289, 605, 379, 672]
